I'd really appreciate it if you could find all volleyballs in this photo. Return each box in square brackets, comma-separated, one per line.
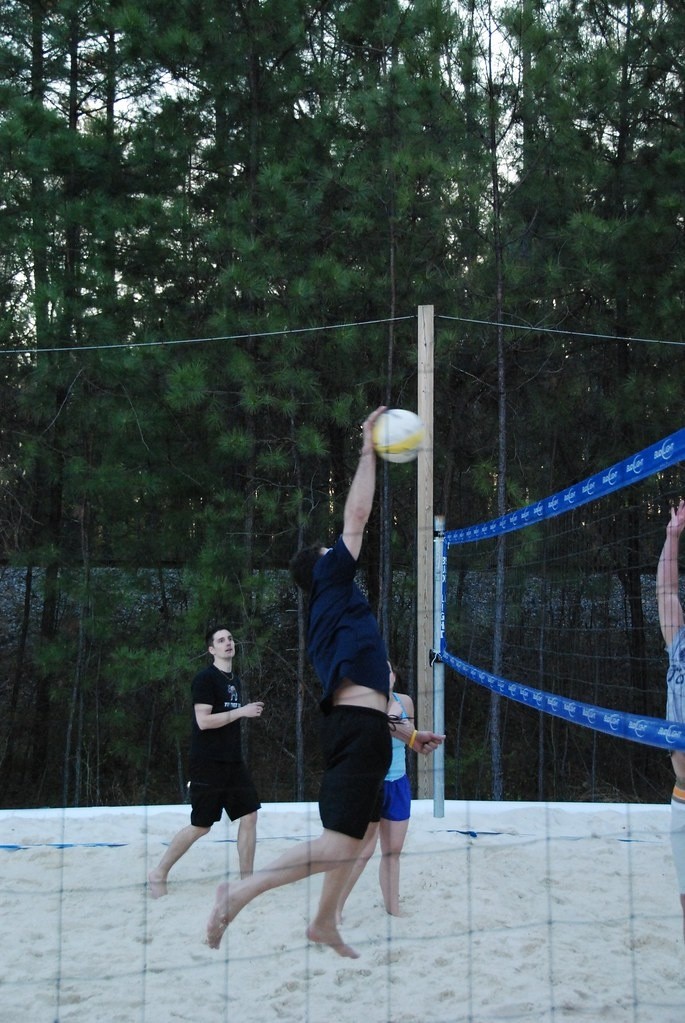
[370, 407, 424, 464]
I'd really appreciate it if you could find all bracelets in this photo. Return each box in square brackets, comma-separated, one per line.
[409, 730, 417, 749]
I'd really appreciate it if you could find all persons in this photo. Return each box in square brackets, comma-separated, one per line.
[149, 626, 262, 897]
[657, 500, 685, 923]
[207, 405, 446, 959]
[334, 660, 415, 925]
